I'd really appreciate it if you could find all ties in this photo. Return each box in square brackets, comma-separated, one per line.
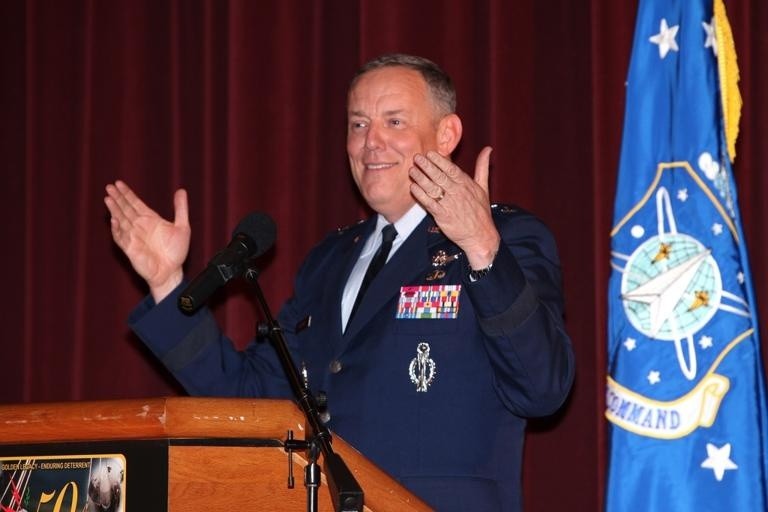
[343, 224, 398, 335]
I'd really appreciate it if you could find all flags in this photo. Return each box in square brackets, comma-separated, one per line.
[607, 0, 767, 512]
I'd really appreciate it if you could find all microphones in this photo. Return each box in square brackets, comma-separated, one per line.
[177, 213, 277, 316]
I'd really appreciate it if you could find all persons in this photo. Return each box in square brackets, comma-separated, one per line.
[105, 54, 573, 512]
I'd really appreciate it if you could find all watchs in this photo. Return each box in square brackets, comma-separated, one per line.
[470, 264, 493, 281]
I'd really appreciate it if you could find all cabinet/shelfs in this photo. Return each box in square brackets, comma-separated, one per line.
[0, 397, 436, 512]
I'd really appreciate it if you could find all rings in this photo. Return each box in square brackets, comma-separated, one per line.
[434, 190, 445, 203]
